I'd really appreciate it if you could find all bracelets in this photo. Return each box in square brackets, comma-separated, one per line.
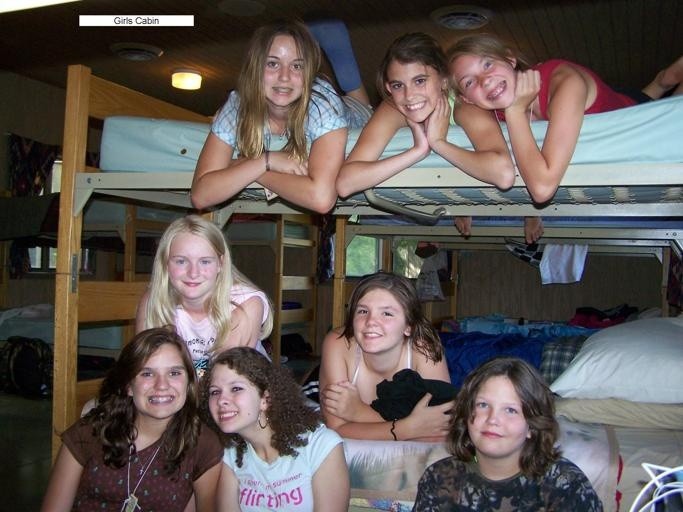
[390, 418, 398, 442]
[264, 150, 272, 173]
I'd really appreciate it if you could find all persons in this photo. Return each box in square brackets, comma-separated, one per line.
[333, 32, 544, 244]
[410, 358, 605, 512]
[446, 27, 682, 206]
[301, 271, 455, 446]
[134, 217, 276, 396]
[188, 12, 376, 219]
[38, 327, 224, 512]
[197, 345, 350, 512]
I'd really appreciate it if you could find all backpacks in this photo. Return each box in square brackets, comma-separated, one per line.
[572, 302, 637, 328]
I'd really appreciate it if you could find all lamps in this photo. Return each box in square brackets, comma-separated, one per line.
[430, 6, 492, 31]
[108, 41, 163, 62]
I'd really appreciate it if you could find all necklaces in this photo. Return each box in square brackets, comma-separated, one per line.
[118, 421, 174, 512]
